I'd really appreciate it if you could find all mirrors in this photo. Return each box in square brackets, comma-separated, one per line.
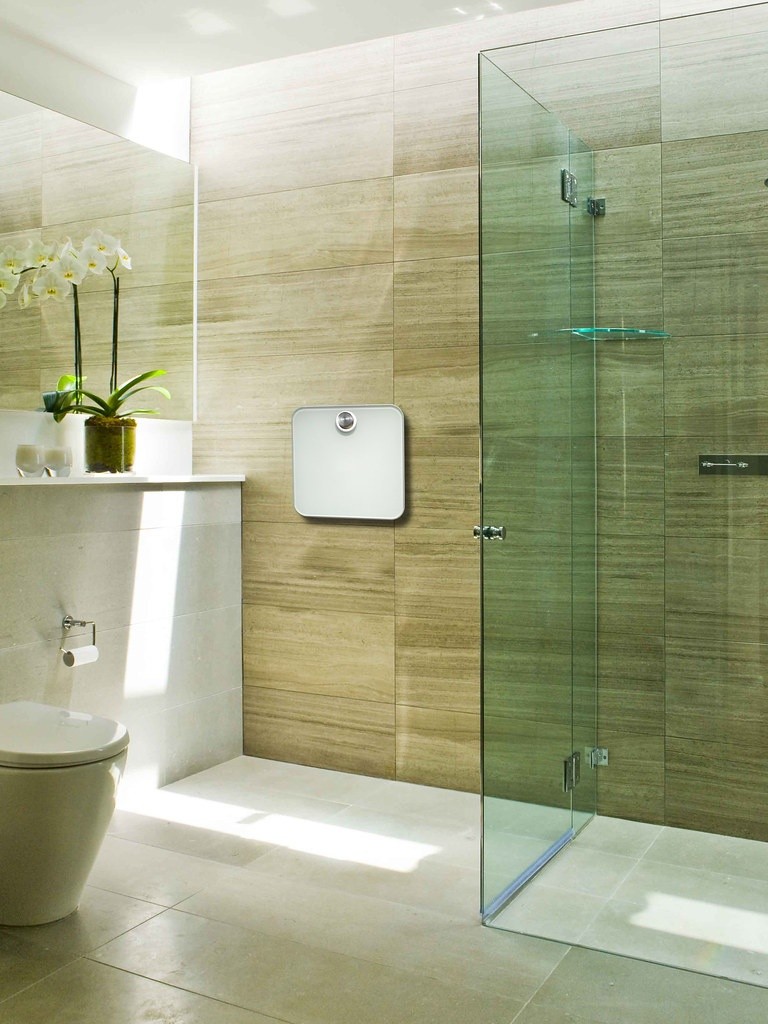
[0, 122, 198, 420]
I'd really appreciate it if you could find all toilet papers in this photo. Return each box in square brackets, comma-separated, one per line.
[64, 645, 101, 665]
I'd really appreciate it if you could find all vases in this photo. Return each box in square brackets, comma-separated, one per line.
[83, 416, 138, 472]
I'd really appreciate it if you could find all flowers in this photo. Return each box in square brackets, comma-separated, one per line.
[48, 234, 172, 419]
[0, 236, 88, 413]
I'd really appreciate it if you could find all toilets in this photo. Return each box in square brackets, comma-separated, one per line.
[0, 703, 133, 924]
